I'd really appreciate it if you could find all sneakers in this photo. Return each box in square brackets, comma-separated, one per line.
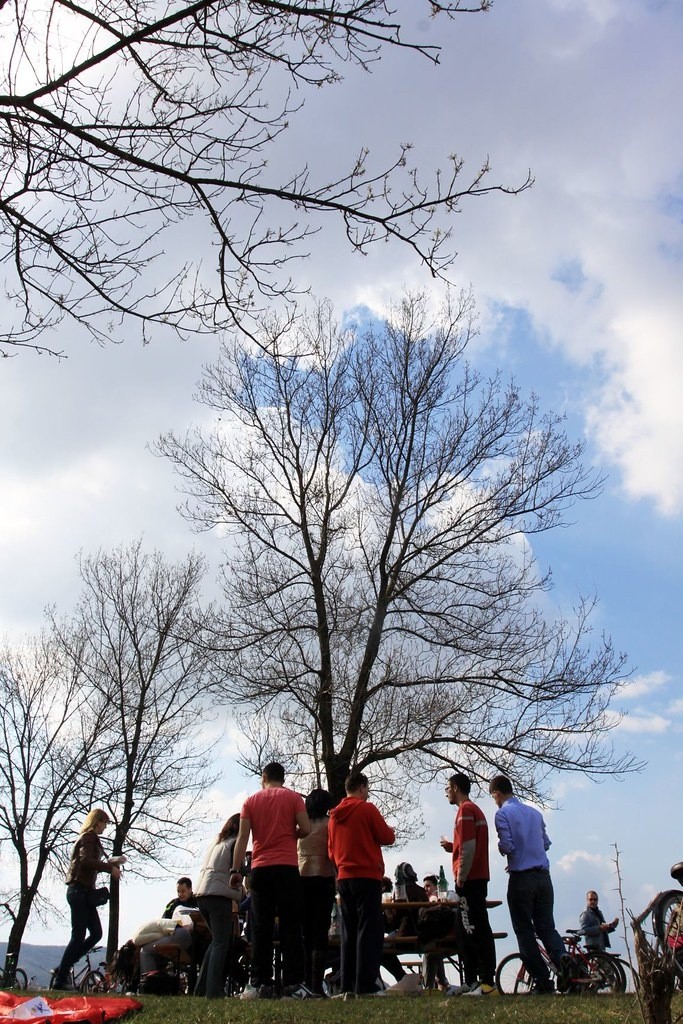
[452, 980, 500, 996]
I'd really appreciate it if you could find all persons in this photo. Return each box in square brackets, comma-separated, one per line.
[229, 762, 311, 998]
[195, 788, 454, 998]
[440, 773, 500, 998]
[106, 919, 191, 989]
[162, 877, 198, 920]
[488, 775, 572, 998]
[330, 774, 394, 999]
[580, 890, 619, 993]
[51, 809, 126, 990]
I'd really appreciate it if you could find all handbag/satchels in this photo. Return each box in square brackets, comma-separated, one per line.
[416, 904, 456, 944]
[386, 974, 423, 993]
[94, 887, 109, 907]
[138, 970, 180, 996]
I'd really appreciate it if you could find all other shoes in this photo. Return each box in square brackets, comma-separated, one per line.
[50, 967, 59, 976]
[284, 984, 323, 1002]
[356, 991, 393, 999]
[255, 984, 273, 1000]
[443, 984, 451, 993]
[343, 992, 356, 1002]
[52, 982, 77, 992]
[557, 953, 572, 992]
[425, 987, 432, 995]
[526, 980, 554, 996]
[322, 979, 331, 998]
[331, 990, 345, 1000]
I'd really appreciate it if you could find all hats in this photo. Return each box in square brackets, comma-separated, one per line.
[395, 862, 417, 880]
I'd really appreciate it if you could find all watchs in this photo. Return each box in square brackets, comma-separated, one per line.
[229, 868, 241, 874]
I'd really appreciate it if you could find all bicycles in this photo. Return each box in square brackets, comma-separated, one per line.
[652, 861, 683, 977]
[50, 946, 129, 992]
[0, 953, 37, 991]
[496, 929, 640, 998]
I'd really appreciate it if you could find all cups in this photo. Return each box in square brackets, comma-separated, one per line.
[428, 895, 437, 902]
[439, 891, 447, 903]
[382, 893, 391, 903]
[440, 835, 448, 844]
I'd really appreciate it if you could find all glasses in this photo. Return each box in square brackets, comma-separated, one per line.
[445, 787, 451, 793]
[590, 898, 597, 901]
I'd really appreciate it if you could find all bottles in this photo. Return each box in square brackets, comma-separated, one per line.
[394, 865, 406, 903]
[247, 855, 253, 881]
[437, 865, 447, 896]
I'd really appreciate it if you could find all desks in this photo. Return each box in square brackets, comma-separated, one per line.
[375, 899, 502, 987]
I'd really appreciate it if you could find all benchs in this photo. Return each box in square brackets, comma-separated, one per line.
[328, 929, 508, 966]
